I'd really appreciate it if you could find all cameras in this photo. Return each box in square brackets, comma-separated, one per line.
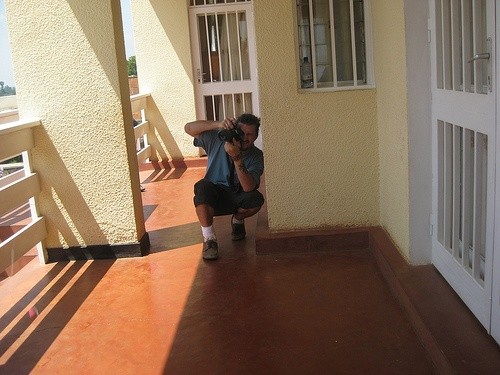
[218, 124, 243, 144]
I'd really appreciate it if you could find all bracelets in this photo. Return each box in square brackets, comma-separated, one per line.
[230, 154, 242, 161]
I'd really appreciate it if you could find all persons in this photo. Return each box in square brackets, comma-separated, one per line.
[184, 114, 264, 259]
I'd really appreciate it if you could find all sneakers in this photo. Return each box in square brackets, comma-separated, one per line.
[202, 234, 219, 260]
[231, 215, 246, 241]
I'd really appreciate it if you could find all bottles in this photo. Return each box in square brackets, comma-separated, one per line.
[301, 57, 313, 87]
